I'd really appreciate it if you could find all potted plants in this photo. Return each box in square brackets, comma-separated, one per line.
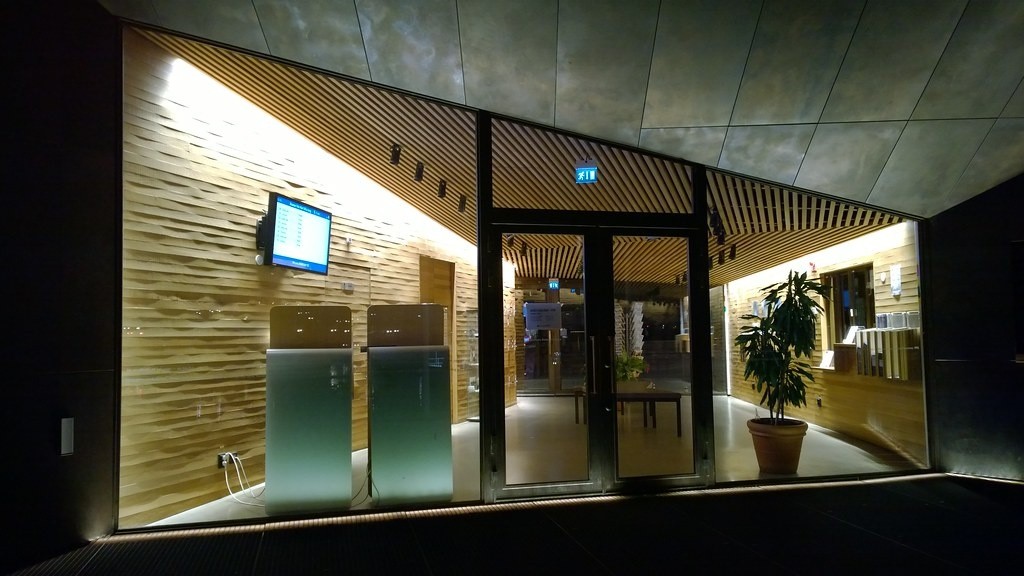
[733, 268, 836, 476]
[616, 352, 649, 379]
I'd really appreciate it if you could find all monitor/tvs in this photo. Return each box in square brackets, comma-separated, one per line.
[264, 192, 332, 275]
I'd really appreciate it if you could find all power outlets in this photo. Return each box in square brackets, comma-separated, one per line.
[218, 451, 238, 469]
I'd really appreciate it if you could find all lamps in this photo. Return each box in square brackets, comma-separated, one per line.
[676, 210, 737, 283]
[389, 146, 527, 255]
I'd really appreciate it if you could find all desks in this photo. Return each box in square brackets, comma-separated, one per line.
[575, 381, 682, 437]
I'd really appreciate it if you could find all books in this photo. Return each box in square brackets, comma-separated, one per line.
[857, 312, 919, 381]
[820, 324, 863, 367]
